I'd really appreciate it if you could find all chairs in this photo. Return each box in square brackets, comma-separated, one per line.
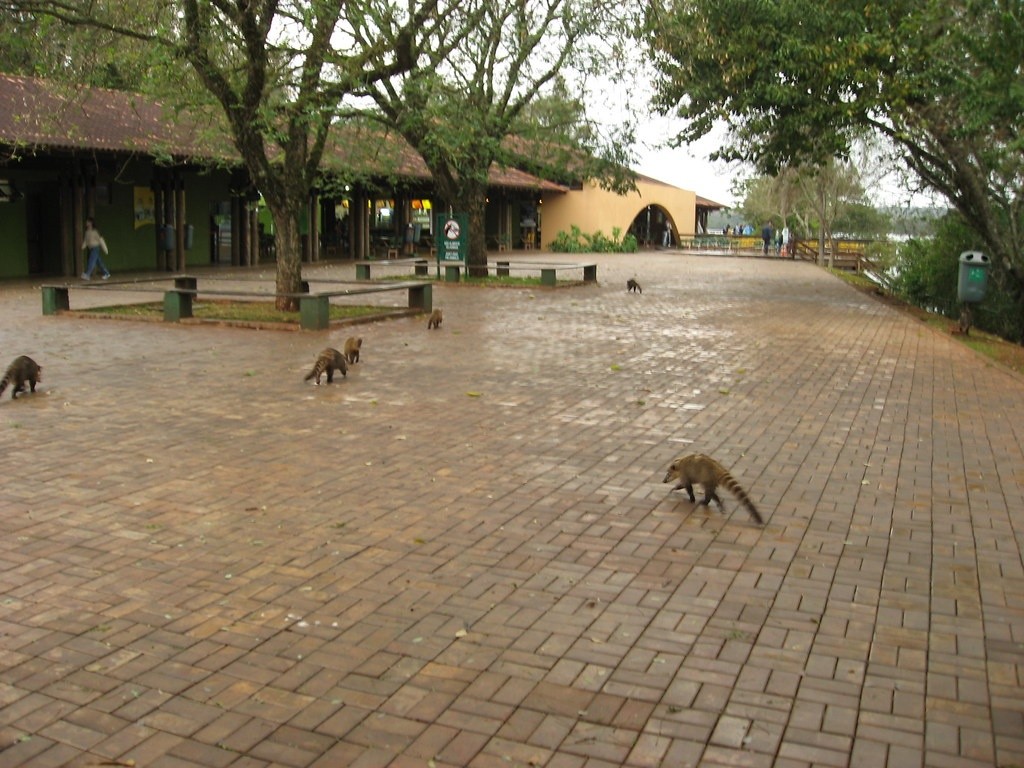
[688, 237, 780, 254]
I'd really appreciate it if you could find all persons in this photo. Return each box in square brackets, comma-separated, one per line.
[660, 219, 676, 248]
[722, 224, 743, 236]
[761, 219, 788, 255]
[80, 216, 111, 280]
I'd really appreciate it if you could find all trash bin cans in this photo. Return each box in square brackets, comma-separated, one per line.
[958, 251, 992, 304]
[162, 223, 174, 251]
[184, 224, 194, 250]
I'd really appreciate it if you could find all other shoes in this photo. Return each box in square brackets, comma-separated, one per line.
[102, 273, 111, 280]
[81, 274, 90, 280]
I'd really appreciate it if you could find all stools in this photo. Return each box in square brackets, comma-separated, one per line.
[430, 246, 437, 256]
[498, 244, 506, 253]
[387, 248, 398, 259]
[524, 243, 531, 251]
